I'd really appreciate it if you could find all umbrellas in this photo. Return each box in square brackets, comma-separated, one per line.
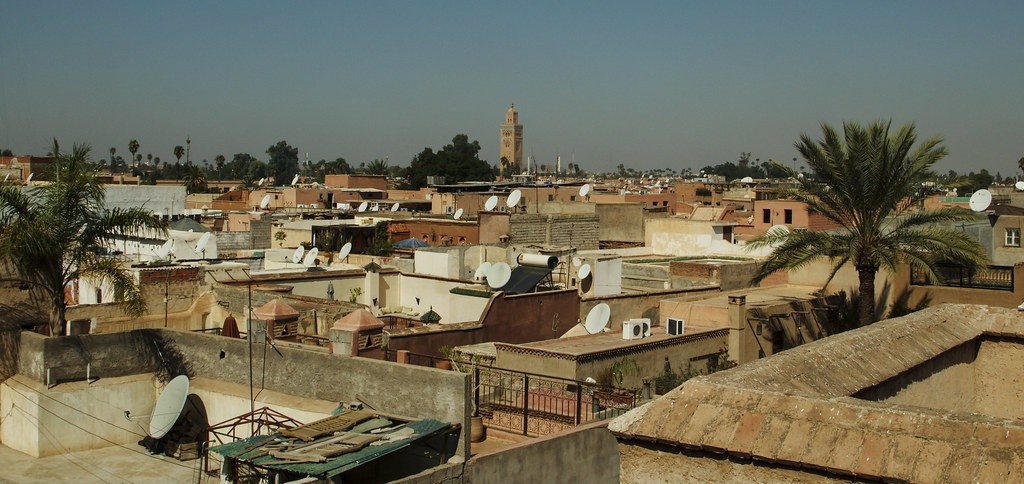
[222, 315, 240, 339]
[392, 237, 430, 258]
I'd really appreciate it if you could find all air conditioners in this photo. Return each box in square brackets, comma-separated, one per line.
[623, 321, 643, 340]
[630, 318, 651, 336]
[666, 317, 684, 336]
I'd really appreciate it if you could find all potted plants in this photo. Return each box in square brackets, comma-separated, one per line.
[599, 357, 633, 409]
[439, 344, 500, 441]
